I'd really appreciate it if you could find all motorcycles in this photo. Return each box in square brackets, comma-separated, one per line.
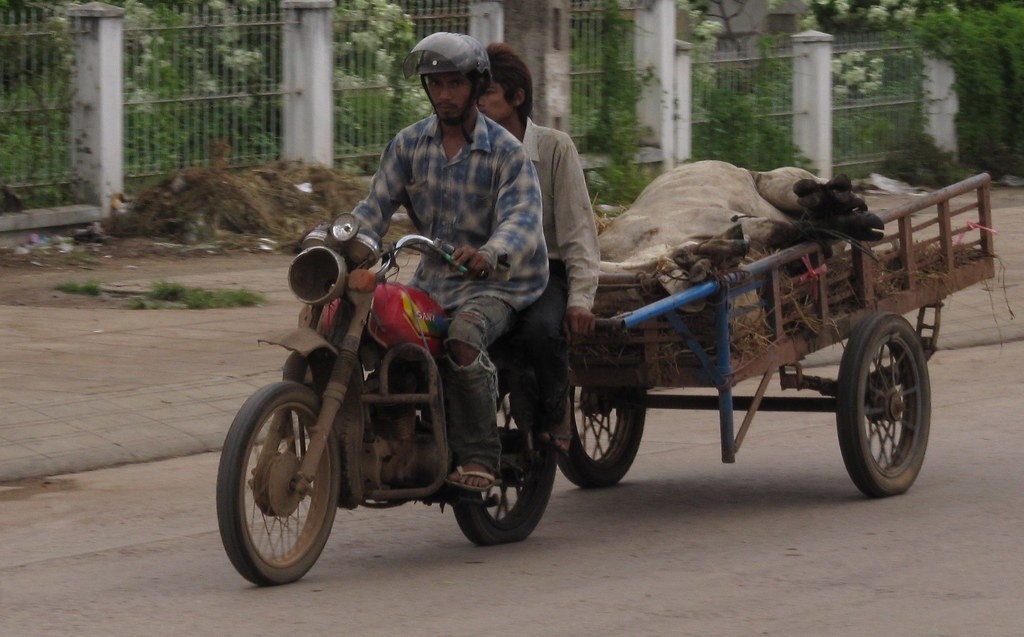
[216, 215, 579, 588]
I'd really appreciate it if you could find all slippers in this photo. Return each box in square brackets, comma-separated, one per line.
[551, 428, 573, 457]
[444, 465, 495, 494]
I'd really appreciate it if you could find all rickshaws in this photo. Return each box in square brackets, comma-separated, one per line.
[547, 166, 1001, 500]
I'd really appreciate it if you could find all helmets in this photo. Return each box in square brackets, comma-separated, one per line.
[418, 33, 493, 93]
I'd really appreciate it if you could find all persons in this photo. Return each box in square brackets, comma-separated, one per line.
[348, 34, 548, 491]
[474, 42, 601, 473]
